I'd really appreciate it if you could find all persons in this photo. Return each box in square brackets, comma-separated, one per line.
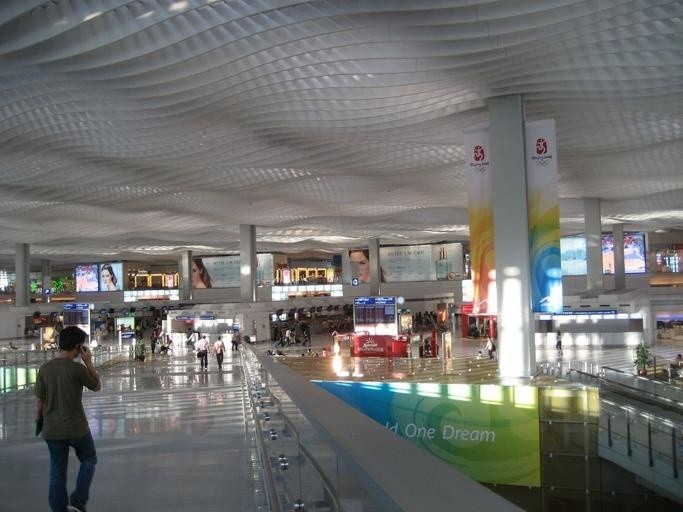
[3, 276, 495, 382]
[348, 250, 369, 283]
[556, 331, 561, 349]
[100, 262, 118, 294]
[33, 326, 101, 511]
[191, 258, 212, 290]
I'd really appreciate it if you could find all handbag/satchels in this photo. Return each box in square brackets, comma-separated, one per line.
[197, 352, 205, 358]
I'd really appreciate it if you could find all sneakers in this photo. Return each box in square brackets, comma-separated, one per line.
[67, 501, 87, 512]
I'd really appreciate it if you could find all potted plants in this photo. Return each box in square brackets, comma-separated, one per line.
[136, 339, 145, 361]
[634, 342, 652, 376]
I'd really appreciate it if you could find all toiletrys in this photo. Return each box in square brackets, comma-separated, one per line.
[435, 246, 451, 282]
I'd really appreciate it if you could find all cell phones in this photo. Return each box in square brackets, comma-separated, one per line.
[78, 345, 86, 353]
